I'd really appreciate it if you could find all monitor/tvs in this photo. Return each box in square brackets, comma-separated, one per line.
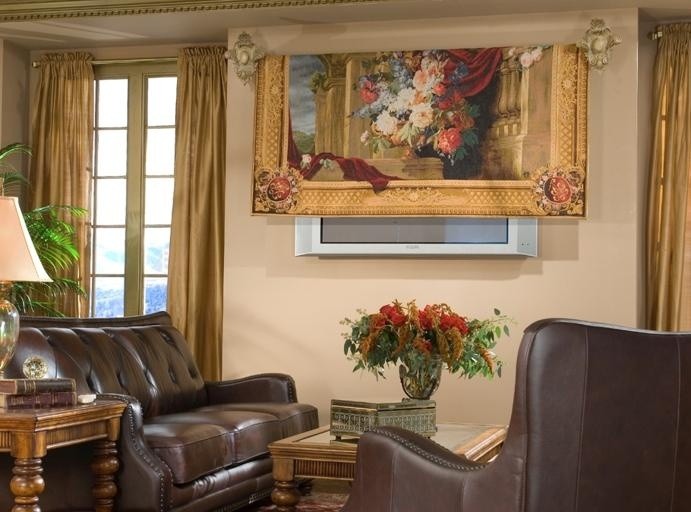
[294, 215, 537, 258]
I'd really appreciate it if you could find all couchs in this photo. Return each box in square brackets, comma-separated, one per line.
[4, 309, 319, 512]
[341, 317, 691, 511]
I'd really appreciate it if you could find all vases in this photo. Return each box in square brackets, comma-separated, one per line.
[398, 350, 443, 398]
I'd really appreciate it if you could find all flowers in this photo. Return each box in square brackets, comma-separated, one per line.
[342, 43, 550, 170]
[338, 297, 521, 397]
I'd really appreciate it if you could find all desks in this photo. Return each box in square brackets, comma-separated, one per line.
[0, 397, 127, 512]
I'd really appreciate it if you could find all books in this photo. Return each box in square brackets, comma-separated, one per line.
[0, 391, 80, 410]
[0, 377, 76, 395]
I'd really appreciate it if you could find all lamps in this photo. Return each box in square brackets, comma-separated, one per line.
[0, 195, 54, 375]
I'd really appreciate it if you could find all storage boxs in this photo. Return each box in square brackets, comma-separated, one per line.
[330, 397, 437, 443]
